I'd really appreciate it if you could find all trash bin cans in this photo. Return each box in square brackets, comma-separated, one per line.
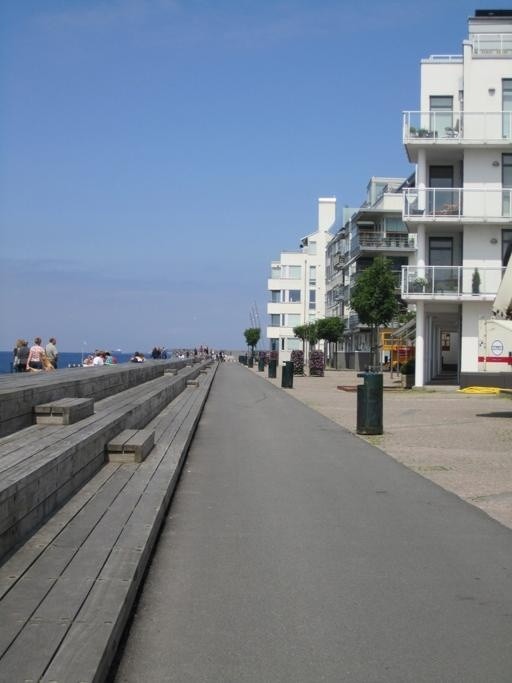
[268, 360, 276, 378]
[357, 372, 383, 435]
[243, 356, 247, 365]
[239, 356, 243, 363]
[248, 357, 253, 368]
[281, 361, 294, 388]
[258, 357, 264, 371]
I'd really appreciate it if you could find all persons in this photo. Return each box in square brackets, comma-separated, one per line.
[82, 337, 225, 366]
[10, 337, 23, 373]
[25, 336, 47, 372]
[12, 339, 30, 372]
[44, 335, 60, 370]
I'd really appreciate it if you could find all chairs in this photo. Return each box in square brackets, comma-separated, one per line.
[444, 118, 460, 138]
